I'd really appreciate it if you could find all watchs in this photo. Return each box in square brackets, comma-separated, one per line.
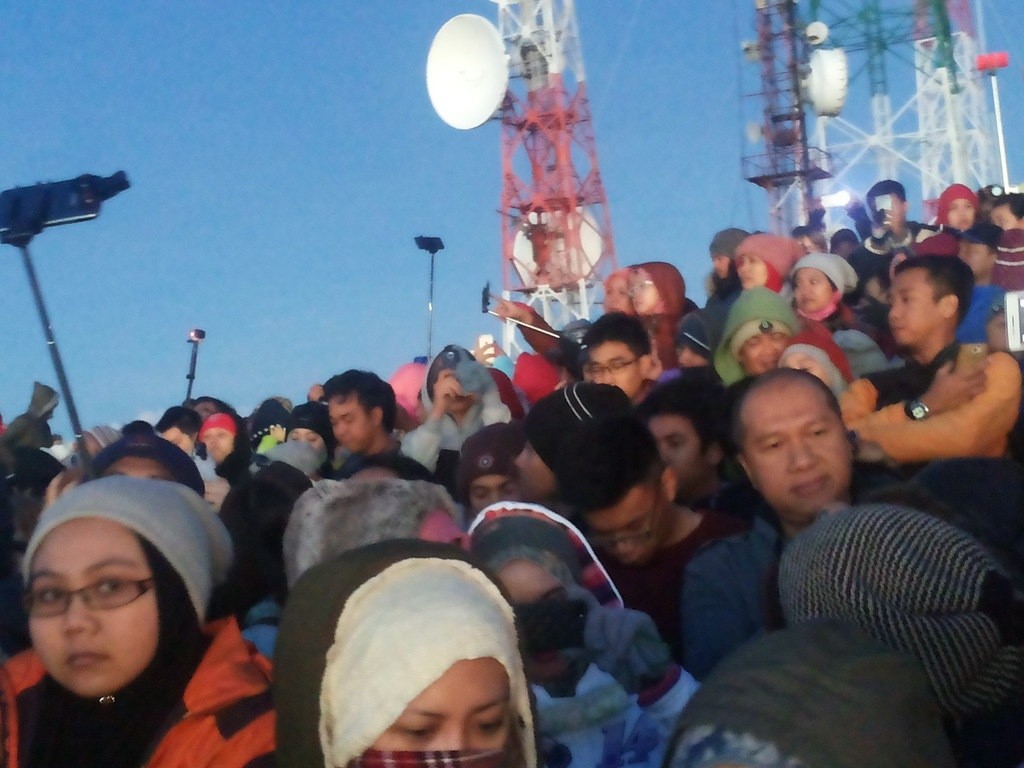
[909, 397, 929, 420]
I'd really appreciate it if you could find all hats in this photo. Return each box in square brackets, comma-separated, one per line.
[953, 223, 1006, 248]
[387, 362, 431, 407]
[18, 473, 238, 631]
[675, 311, 711, 361]
[909, 232, 961, 261]
[709, 228, 753, 262]
[728, 318, 797, 363]
[732, 232, 807, 281]
[460, 420, 527, 476]
[990, 228, 1024, 291]
[81, 433, 206, 499]
[935, 183, 980, 225]
[470, 514, 581, 594]
[283, 400, 336, 463]
[788, 251, 860, 298]
[525, 380, 638, 503]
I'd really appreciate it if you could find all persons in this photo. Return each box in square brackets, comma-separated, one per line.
[1, 180, 1023, 768]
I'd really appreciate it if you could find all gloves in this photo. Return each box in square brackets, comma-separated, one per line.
[565, 584, 672, 696]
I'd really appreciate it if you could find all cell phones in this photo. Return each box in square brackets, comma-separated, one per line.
[875, 195, 892, 224]
[1004, 289, 1024, 352]
[479, 335, 495, 362]
[956, 344, 987, 372]
[192, 329, 205, 338]
[486, 282, 490, 305]
[414, 237, 444, 250]
[517, 600, 588, 647]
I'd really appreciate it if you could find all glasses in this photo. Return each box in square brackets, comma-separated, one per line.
[577, 483, 661, 549]
[21, 574, 158, 618]
[626, 279, 654, 297]
[586, 351, 644, 374]
